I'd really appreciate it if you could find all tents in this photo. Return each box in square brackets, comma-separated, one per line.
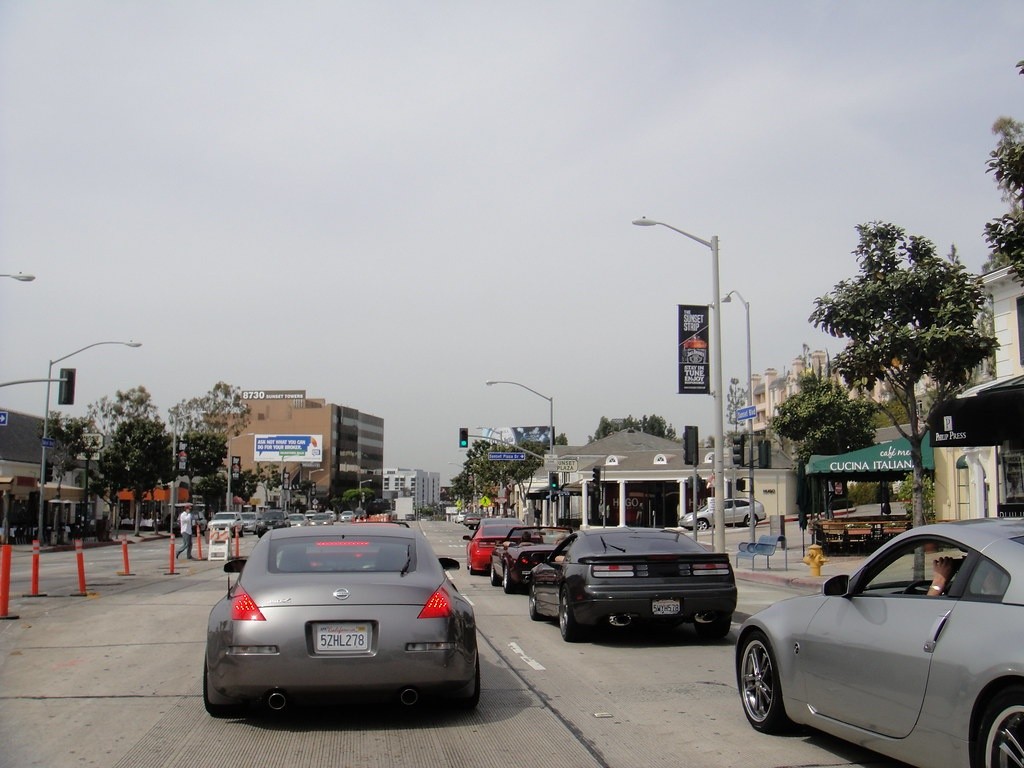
[804, 429, 936, 520]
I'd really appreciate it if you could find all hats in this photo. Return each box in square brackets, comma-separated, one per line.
[184, 505, 193, 510]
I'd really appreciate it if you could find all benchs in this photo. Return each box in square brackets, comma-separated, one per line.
[736, 535, 787, 572]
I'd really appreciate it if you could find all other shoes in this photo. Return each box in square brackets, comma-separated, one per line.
[175, 553, 178, 559]
[189, 556, 194, 559]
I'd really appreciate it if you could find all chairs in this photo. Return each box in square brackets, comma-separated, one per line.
[334, 553, 361, 571]
[280, 549, 309, 572]
[376, 544, 405, 571]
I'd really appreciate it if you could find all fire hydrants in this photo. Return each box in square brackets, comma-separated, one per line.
[803, 543, 828, 576]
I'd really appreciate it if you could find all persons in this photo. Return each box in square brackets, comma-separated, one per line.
[503, 531, 531, 547]
[555, 555, 565, 561]
[556, 536, 571, 546]
[927, 546, 969, 597]
[636, 502, 644, 522]
[176, 505, 195, 560]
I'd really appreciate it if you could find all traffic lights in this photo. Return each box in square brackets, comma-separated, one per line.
[682, 425, 698, 465]
[549, 473, 559, 490]
[551, 494, 559, 502]
[593, 466, 601, 486]
[459, 427, 469, 448]
[732, 434, 745, 467]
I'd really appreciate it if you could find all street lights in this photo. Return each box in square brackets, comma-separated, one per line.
[629, 218, 725, 559]
[485, 380, 553, 456]
[170, 410, 207, 539]
[37, 339, 141, 547]
[227, 432, 255, 513]
[358, 479, 372, 507]
[279, 452, 331, 515]
[721, 288, 755, 544]
[446, 426, 503, 523]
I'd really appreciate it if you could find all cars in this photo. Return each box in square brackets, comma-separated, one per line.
[462, 514, 481, 526]
[733, 517, 1024, 768]
[455, 512, 469, 524]
[205, 508, 368, 538]
[527, 525, 739, 643]
[678, 497, 766, 531]
[462, 519, 547, 575]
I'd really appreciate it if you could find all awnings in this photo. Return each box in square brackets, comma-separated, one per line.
[0, 474, 85, 502]
[927, 375, 1024, 448]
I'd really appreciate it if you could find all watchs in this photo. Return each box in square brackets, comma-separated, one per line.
[930, 586, 944, 594]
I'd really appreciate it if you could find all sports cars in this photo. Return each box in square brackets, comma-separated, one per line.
[202, 524, 480, 714]
[488, 526, 576, 594]
